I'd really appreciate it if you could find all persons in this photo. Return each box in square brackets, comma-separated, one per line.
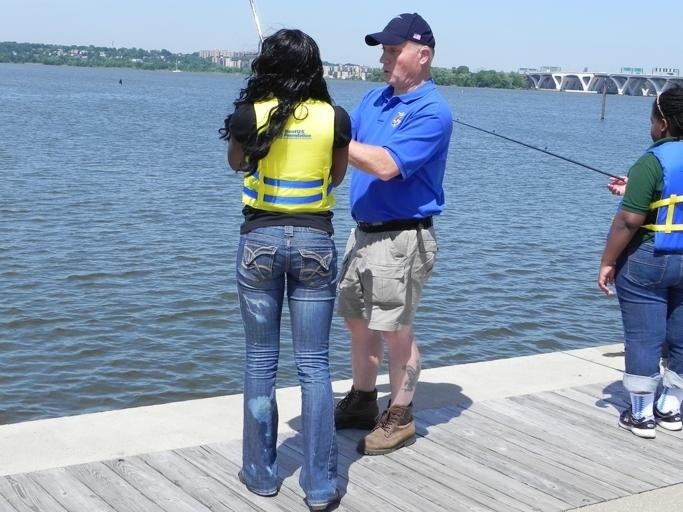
[218, 26, 352, 512]
[334, 11, 451, 455]
[598, 84, 683, 440]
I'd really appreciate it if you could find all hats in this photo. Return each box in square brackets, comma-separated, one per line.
[365, 13, 435, 48]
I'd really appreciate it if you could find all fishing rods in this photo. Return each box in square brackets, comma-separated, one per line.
[452, 119, 624, 182]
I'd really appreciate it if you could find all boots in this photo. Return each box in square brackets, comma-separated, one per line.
[335, 386, 379, 430]
[356, 398, 416, 455]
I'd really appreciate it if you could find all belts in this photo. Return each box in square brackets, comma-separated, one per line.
[358, 217, 432, 233]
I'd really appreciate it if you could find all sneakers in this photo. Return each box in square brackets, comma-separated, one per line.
[653, 394, 683, 431]
[618, 405, 657, 438]
[238, 471, 278, 497]
[311, 488, 340, 512]
